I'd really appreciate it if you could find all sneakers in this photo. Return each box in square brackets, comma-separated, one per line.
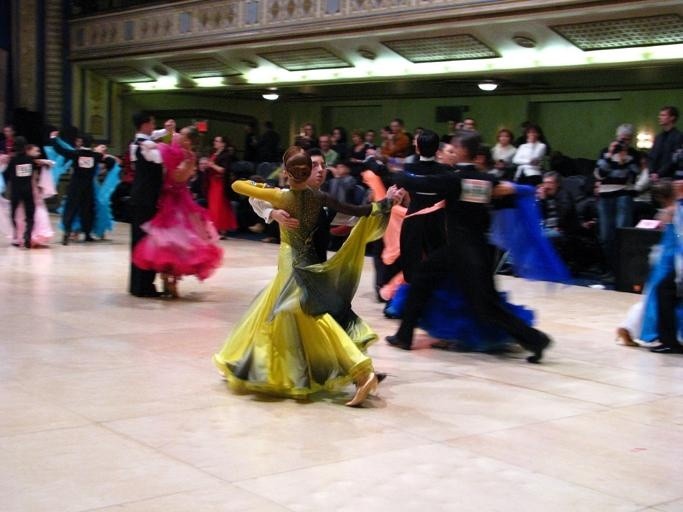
[248, 223, 274, 243]
[330, 225, 352, 236]
[526, 334, 549, 362]
[387, 336, 411, 350]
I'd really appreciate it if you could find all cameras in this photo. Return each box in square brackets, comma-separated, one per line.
[612, 142, 623, 155]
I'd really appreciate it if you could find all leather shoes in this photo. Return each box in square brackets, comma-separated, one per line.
[618, 327, 638, 346]
[345, 372, 386, 407]
[650, 342, 683, 354]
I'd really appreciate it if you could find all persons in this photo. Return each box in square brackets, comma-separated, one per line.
[213, 146, 405, 406]
[128, 111, 223, 300]
[180, 118, 591, 276]
[593, 107, 683, 355]
[366, 129, 552, 362]
[249, 148, 406, 388]
[0, 127, 123, 250]
